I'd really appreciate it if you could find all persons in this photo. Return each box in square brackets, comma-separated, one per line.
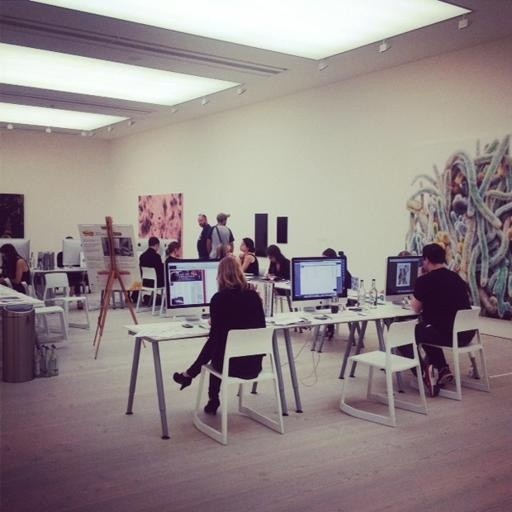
[195, 214, 211, 259]
[206, 212, 235, 260]
[397, 241, 477, 399]
[398, 251, 411, 256]
[319, 247, 356, 341]
[0, 242, 32, 296]
[238, 237, 259, 275]
[139, 235, 166, 288]
[166, 240, 185, 263]
[173, 254, 266, 414]
[265, 244, 292, 296]
[55, 235, 84, 311]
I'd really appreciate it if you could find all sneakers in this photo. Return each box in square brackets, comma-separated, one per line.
[437, 365, 454, 387]
[423, 363, 439, 398]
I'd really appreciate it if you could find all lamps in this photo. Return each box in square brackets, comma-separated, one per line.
[317, 12, 473, 76]
[88, 119, 136, 137]
[170, 83, 246, 115]
[6, 122, 88, 138]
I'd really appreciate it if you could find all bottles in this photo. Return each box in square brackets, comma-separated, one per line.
[368, 278, 377, 309]
[331, 289, 339, 313]
[359, 279, 366, 305]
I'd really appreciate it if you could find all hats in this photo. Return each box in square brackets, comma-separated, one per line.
[217, 213, 230, 220]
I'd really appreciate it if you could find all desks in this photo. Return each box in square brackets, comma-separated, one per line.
[247, 275, 294, 313]
[30, 266, 89, 310]
[1, 284, 44, 311]
[368, 290, 484, 396]
[123, 319, 293, 439]
[270, 301, 407, 415]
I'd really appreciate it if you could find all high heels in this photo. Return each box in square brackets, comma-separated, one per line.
[204, 398, 220, 415]
[173, 372, 192, 391]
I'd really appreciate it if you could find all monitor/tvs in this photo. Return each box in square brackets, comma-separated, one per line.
[0, 238, 30, 269]
[290, 256, 347, 315]
[62, 239, 82, 269]
[385, 256, 427, 309]
[165, 258, 222, 325]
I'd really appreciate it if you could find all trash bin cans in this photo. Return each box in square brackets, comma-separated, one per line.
[0, 304, 36, 383]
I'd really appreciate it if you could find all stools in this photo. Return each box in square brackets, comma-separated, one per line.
[33, 306, 71, 350]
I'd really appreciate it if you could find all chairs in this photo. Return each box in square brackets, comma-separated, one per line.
[137, 265, 164, 314]
[194, 326, 286, 446]
[338, 319, 431, 428]
[41, 272, 92, 332]
[405, 305, 490, 401]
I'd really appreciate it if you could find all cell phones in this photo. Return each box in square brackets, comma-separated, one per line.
[183, 323, 194, 328]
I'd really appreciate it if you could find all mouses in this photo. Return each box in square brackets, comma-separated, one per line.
[297, 318, 310, 325]
